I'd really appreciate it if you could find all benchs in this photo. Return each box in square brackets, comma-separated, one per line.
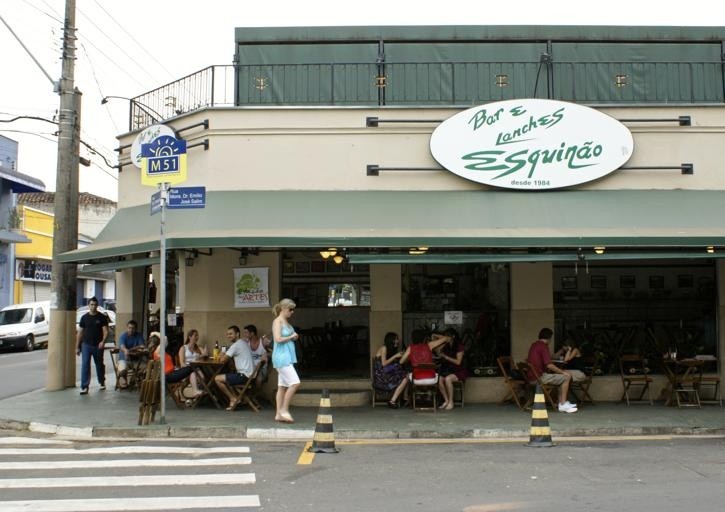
[296, 325, 372, 365]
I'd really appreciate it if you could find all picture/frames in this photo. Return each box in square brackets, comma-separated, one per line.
[619, 275, 636, 288]
[590, 275, 608, 289]
[678, 273, 694, 288]
[560, 276, 577, 290]
[649, 275, 664, 289]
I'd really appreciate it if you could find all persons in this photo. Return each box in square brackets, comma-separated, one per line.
[214, 325, 257, 412]
[147, 331, 209, 398]
[271, 298, 301, 422]
[76, 298, 109, 394]
[371, 332, 410, 408]
[238, 324, 272, 407]
[399, 330, 448, 401]
[555, 337, 586, 383]
[438, 328, 467, 410]
[114, 320, 146, 392]
[178, 329, 209, 402]
[528, 328, 578, 413]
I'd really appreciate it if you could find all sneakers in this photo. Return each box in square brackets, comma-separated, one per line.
[99, 385, 105, 389]
[79, 388, 88, 394]
[225, 398, 241, 411]
[388, 401, 397, 409]
[558, 403, 577, 413]
[439, 402, 447, 409]
[445, 403, 454, 410]
[566, 401, 577, 407]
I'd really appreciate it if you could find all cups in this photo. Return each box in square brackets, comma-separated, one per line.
[193, 353, 200, 360]
[222, 347, 226, 352]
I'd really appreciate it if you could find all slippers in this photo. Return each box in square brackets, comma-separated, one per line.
[192, 390, 209, 397]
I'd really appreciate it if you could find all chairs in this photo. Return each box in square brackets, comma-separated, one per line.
[495, 350, 605, 408]
[370, 347, 469, 412]
[108, 347, 270, 428]
[616, 352, 725, 409]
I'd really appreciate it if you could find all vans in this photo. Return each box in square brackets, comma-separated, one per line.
[0, 300, 52, 352]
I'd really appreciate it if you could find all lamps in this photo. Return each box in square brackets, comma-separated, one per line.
[238, 246, 248, 267]
[318, 247, 344, 264]
[594, 246, 605, 254]
[185, 248, 199, 267]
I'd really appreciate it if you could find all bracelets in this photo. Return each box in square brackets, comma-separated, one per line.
[446, 356, 448, 360]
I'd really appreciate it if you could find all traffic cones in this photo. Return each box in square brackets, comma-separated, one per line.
[306, 385, 342, 454]
[520, 383, 559, 449]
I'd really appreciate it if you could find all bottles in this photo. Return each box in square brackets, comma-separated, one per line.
[213, 340, 219, 359]
[203, 344, 208, 359]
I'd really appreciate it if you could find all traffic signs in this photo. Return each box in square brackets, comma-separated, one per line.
[151, 190, 162, 215]
[167, 185, 205, 209]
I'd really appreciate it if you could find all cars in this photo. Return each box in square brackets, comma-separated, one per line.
[76, 310, 116, 346]
[78, 305, 105, 311]
